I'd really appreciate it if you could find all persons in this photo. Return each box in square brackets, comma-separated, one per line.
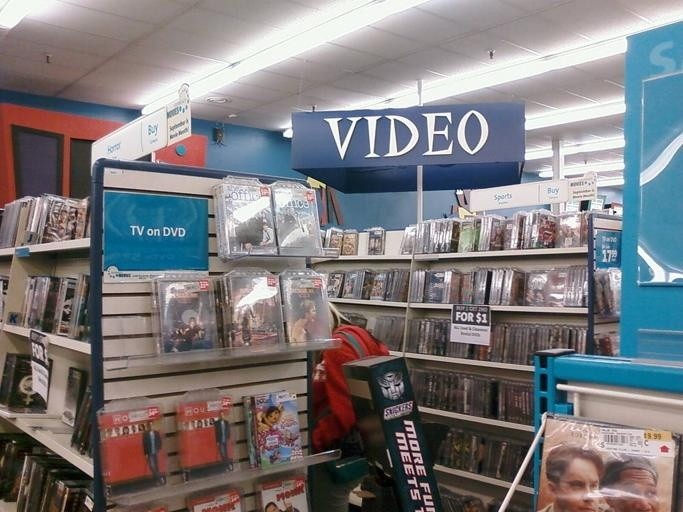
[600, 453, 658, 512]
[289, 297, 318, 347]
[309, 303, 388, 511]
[263, 497, 300, 512]
[214, 409, 231, 466]
[140, 421, 165, 483]
[539, 439, 607, 512]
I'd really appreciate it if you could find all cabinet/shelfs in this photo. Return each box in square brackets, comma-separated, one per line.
[1, 158, 343, 512]
[307, 205, 622, 511]
